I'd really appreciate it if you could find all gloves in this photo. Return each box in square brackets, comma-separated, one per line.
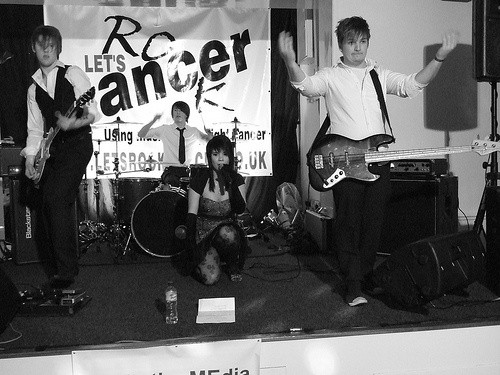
[221, 164, 245, 215]
[183, 213, 197, 273]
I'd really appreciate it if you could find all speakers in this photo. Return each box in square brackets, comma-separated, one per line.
[7, 179, 55, 264]
[424, 42, 478, 130]
[377, 171, 458, 255]
[473, 0, 500, 83]
[0, 144, 24, 178]
[0, 262, 22, 333]
[372, 232, 491, 314]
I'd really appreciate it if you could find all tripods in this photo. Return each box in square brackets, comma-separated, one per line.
[77, 154, 135, 255]
[473, 82, 500, 296]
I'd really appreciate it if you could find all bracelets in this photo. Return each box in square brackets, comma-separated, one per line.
[434, 55, 447, 63]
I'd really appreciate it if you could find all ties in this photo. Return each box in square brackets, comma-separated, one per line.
[175, 127, 186, 165]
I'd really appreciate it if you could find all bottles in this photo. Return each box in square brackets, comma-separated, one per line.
[165, 281, 178, 325]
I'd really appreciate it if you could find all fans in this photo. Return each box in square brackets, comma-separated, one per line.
[275, 182, 305, 230]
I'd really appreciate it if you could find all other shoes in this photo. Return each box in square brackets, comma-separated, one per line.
[231, 272, 243, 282]
[39, 279, 74, 290]
[348, 296, 369, 308]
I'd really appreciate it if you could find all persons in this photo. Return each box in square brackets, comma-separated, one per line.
[277, 17, 459, 306]
[138, 101, 212, 190]
[19, 25, 102, 295]
[185, 135, 248, 284]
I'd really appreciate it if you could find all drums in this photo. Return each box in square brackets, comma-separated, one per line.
[130, 185, 189, 258]
[115, 177, 165, 227]
[188, 163, 210, 178]
[178, 177, 191, 192]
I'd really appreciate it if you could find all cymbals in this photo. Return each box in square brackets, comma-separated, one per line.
[211, 122, 256, 126]
[93, 119, 145, 125]
[127, 160, 178, 164]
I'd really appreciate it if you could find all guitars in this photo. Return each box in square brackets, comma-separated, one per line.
[31, 85, 95, 184]
[307, 133, 500, 193]
